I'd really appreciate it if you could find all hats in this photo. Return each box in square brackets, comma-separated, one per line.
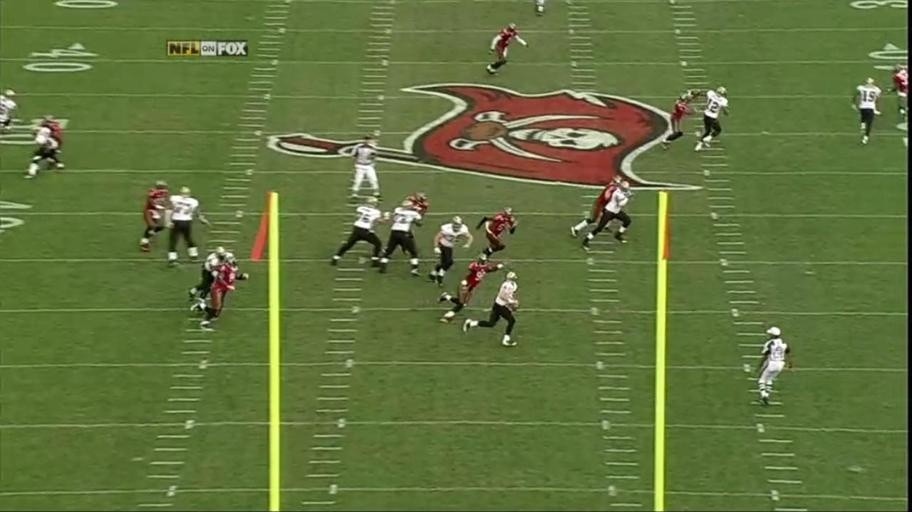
[506, 272, 518, 279]
[215, 247, 224, 255]
[766, 326, 781, 336]
[479, 253, 488, 261]
[503, 207, 513, 215]
[865, 77, 875, 83]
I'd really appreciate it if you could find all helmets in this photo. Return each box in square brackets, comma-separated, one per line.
[716, 87, 727, 94]
[612, 175, 630, 192]
[366, 192, 428, 208]
[452, 215, 462, 225]
[224, 253, 235, 264]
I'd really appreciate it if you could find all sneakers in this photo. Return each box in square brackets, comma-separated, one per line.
[860, 122, 866, 130]
[463, 318, 472, 334]
[571, 225, 628, 251]
[428, 273, 436, 281]
[760, 395, 769, 406]
[330, 257, 338, 266]
[437, 291, 447, 304]
[140, 230, 199, 269]
[23, 162, 65, 180]
[189, 287, 206, 302]
[410, 269, 421, 277]
[190, 303, 203, 312]
[200, 323, 215, 330]
[661, 135, 711, 152]
[485, 66, 499, 75]
[502, 340, 518, 347]
[862, 135, 870, 145]
[440, 316, 449, 324]
[437, 275, 443, 284]
[370, 256, 389, 274]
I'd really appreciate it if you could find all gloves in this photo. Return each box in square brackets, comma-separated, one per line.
[460, 279, 468, 286]
[463, 243, 470, 250]
[433, 247, 442, 255]
[508, 298, 518, 305]
[242, 273, 250, 280]
[211, 270, 219, 278]
[227, 285, 236, 291]
[496, 264, 505, 270]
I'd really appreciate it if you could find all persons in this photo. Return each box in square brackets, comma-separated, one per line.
[667, 84, 699, 144]
[189, 253, 249, 328]
[0, 86, 18, 135]
[851, 76, 885, 145]
[186, 246, 226, 303]
[329, 192, 522, 347]
[485, 24, 526, 76]
[24, 126, 64, 180]
[692, 86, 731, 150]
[568, 175, 623, 239]
[347, 133, 381, 206]
[581, 180, 633, 253]
[887, 63, 910, 123]
[164, 186, 213, 265]
[752, 326, 792, 406]
[141, 179, 168, 253]
[37, 116, 63, 150]
